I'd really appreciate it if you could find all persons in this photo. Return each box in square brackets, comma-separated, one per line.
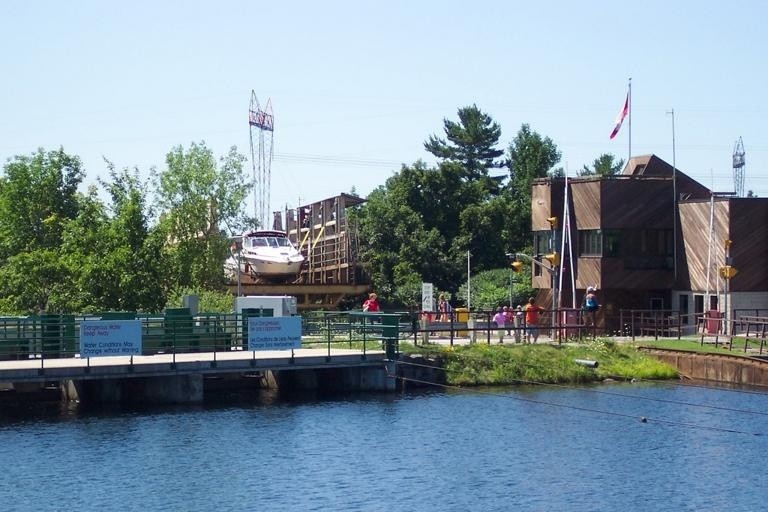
[362, 293, 386, 335]
[425, 293, 437, 336]
[520, 296, 547, 343]
[579, 288, 598, 337]
[493, 304, 524, 344]
[583, 285, 599, 337]
[436, 293, 449, 338]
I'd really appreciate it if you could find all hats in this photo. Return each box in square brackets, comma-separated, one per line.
[587, 287, 597, 292]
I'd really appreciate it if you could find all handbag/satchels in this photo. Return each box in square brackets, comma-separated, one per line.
[363, 300, 369, 312]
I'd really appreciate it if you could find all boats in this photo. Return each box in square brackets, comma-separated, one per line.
[224, 229, 305, 278]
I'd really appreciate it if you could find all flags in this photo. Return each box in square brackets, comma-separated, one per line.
[608, 90, 630, 140]
